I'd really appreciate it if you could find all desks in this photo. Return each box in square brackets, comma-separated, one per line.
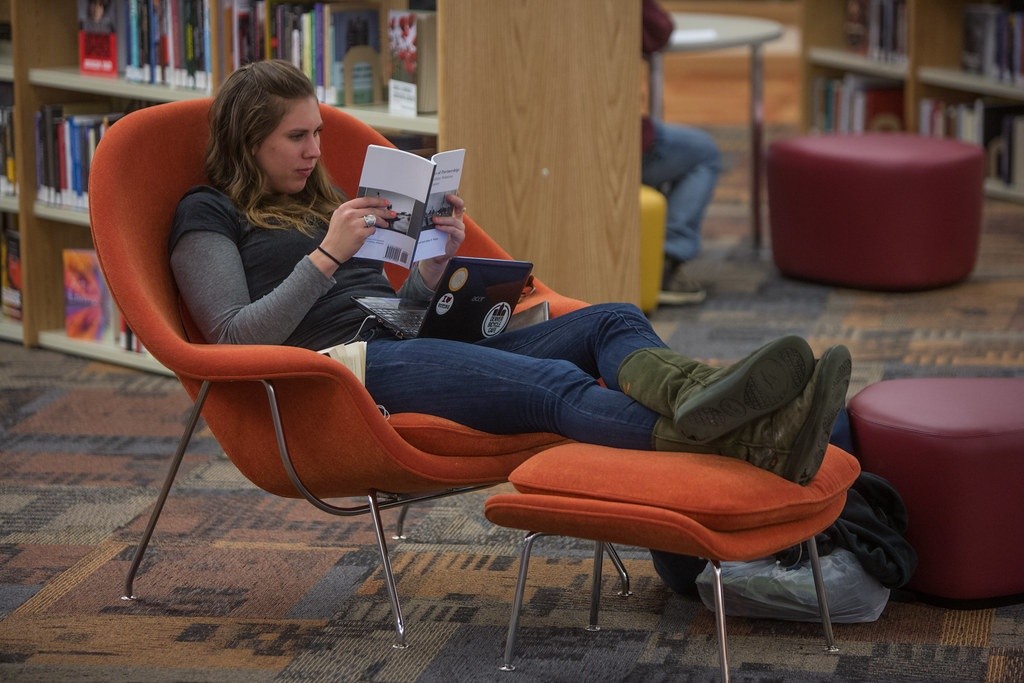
[640, 11, 784, 252]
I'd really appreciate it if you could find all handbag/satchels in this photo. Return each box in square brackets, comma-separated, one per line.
[695, 554, 889, 624]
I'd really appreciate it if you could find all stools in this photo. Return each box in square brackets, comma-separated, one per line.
[483, 487, 838, 683]
[769, 132, 985, 295]
[638, 183, 667, 315]
[846, 376, 1024, 605]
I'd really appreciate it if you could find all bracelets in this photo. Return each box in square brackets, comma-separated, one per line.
[318, 246, 342, 265]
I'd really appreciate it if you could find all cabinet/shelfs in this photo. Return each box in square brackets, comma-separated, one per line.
[799, 0, 1024, 204]
[0, 0, 643, 378]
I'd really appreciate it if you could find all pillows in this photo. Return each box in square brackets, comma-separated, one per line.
[506, 443, 861, 533]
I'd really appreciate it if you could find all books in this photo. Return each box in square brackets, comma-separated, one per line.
[352, 144, 465, 270]
[812, 1, 1024, 187]
[0, 1, 437, 353]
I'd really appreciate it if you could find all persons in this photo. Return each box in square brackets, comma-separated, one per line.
[168, 58, 852, 486]
[642, 114, 719, 304]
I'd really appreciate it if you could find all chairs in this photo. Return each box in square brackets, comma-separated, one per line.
[86, 94, 616, 649]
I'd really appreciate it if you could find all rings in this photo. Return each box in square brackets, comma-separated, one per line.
[364, 215, 376, 228]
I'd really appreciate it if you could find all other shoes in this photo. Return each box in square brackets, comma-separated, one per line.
[658, 262, 706, 304]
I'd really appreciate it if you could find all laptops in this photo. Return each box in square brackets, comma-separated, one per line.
[351, 255, 533, 340]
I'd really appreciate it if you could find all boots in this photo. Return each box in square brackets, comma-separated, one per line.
[652, 345, 852, 487]
[616, 335, 816, 443]
